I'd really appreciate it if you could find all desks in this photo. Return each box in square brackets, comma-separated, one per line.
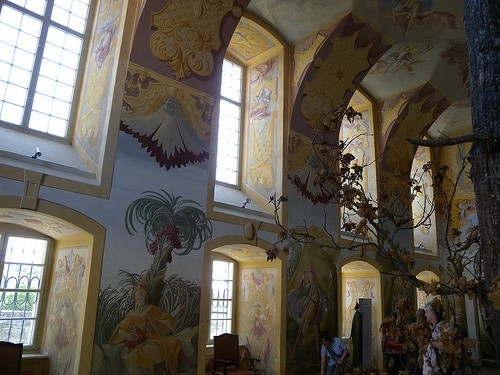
[21, 353, 49, 375]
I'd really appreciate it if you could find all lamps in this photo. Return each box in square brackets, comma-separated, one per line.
[242, 198, 251, 208]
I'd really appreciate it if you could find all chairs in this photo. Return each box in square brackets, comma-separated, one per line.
[0, 341, 23, 375]
[212, 333, 260, 375]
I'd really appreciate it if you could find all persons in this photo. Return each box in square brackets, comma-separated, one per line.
[380, 309, 440, 375]
[321, 331, 348, 375]
[425, 298, 441, 311]
